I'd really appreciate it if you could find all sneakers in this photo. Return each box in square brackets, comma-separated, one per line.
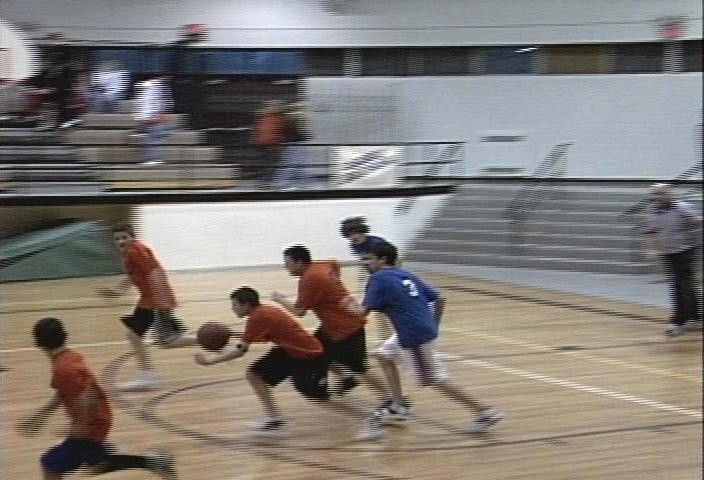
[468, 409, 501, 437]
[348, 427, 387, 449]
[120, 376, 157, 393]
[248, 420, 290, 440]
[664, 315, 703, 337]
[377, 396, 412, 414]
[151, 453, 178, 480]
[332, 375, 361, 403]
[371, 403, 406, 425]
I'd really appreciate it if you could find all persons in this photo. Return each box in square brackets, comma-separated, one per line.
[640, 181, 704, 335]
[192, 285, 383, 441]
[21, 317, 179, 480]
[339, 243, 505, 435]
[338, 214, 403, 282]
[270, 243, 413, 410]
[19, 58, 305, 179]
[92, 221, 199, 389]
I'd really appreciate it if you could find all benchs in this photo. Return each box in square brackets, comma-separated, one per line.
[0, 105, 238, 194]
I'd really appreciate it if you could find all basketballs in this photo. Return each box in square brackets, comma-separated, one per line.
[196, 320, 232, 353]
